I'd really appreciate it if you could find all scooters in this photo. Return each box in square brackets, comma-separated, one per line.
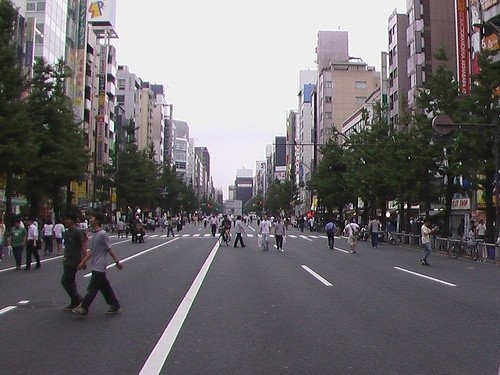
[136, 223, 144, 243]
[377, 229, 396, 245]
[357, 225, 369, 242]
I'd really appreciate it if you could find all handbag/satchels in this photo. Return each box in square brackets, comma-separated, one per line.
[257, 234, 263, 247]
[352, 229, 359, 235]
[327, 229, 334, 236]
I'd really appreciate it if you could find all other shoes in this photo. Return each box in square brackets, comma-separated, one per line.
[234, 246, 239, 248]
[423, 262, 429, 266]
[242, 245, 247, 248]
[72, 308, 89, 315]
[16, 266, 20, 270]
[25, 267, 31, 270]
[105, 306, 121, 314]
[35, 264, 41, 269]
[420, 259, 426, 264]
[64, 306, 76, 310]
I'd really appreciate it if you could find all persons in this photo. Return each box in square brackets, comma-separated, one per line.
[60, 211, 88, 311]
[72, 214, 124, 317]
[0, 213, 486, 269]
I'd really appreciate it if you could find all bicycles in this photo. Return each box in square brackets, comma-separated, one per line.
[447, 237, 479, 261]
[218, 225, 231, 247]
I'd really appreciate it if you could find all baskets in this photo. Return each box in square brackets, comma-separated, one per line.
[219, 228, 224, 233]
